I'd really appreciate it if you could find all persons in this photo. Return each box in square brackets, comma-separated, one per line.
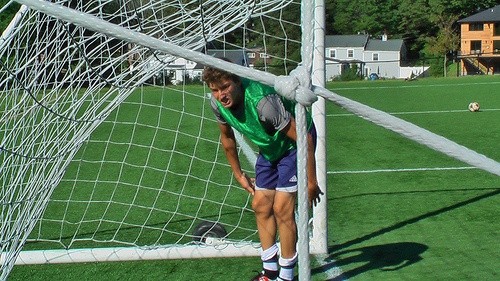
[200, 56, 325, 281]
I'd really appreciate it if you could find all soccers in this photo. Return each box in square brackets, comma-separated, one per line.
[468, 101, 480, 112]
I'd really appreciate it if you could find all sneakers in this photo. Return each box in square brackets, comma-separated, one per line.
[249, 272, 272, 280]
